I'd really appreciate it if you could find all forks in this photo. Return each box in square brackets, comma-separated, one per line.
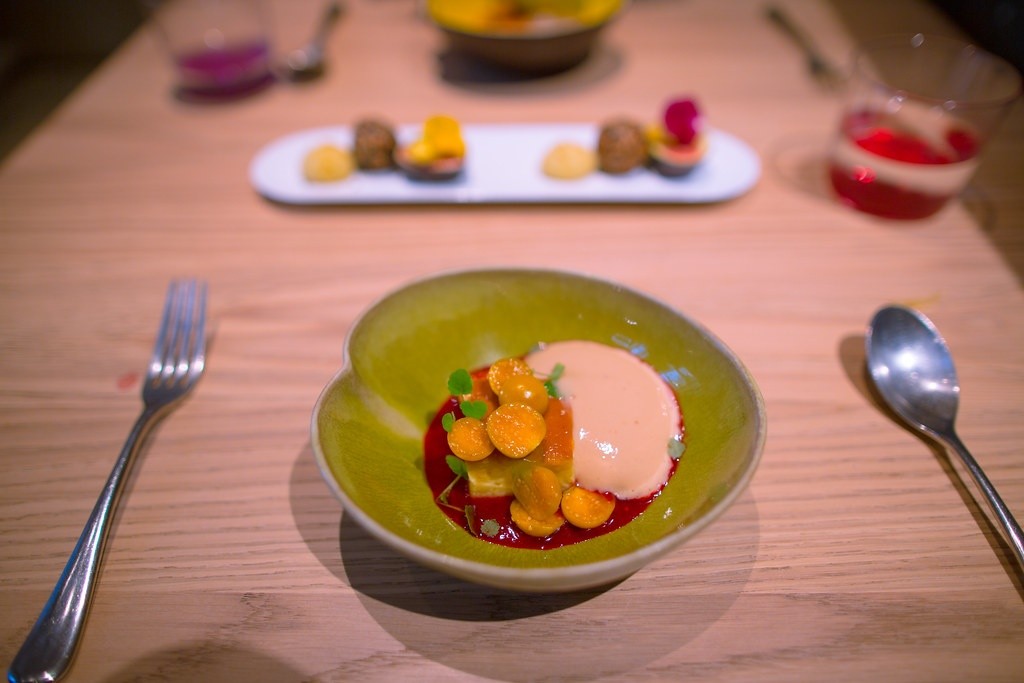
[7, 282, 205, 682]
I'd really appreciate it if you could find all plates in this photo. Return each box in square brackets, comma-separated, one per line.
[251, 122, 758, 204]
[314, 273, 761, 592]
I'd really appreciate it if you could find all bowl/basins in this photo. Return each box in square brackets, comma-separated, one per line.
[415, 0, 623, 76]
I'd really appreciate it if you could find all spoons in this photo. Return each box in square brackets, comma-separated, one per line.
[866, 305, 1024, 572]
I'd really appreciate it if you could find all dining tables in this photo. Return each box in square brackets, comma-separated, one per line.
[1, 0, 1024, 683]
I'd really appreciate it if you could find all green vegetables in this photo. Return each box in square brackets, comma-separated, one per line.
[436, 363, 565, 539]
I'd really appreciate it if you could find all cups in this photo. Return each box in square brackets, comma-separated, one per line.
[144, 0, 275, 100]
[832, 35, 1018, 220]
